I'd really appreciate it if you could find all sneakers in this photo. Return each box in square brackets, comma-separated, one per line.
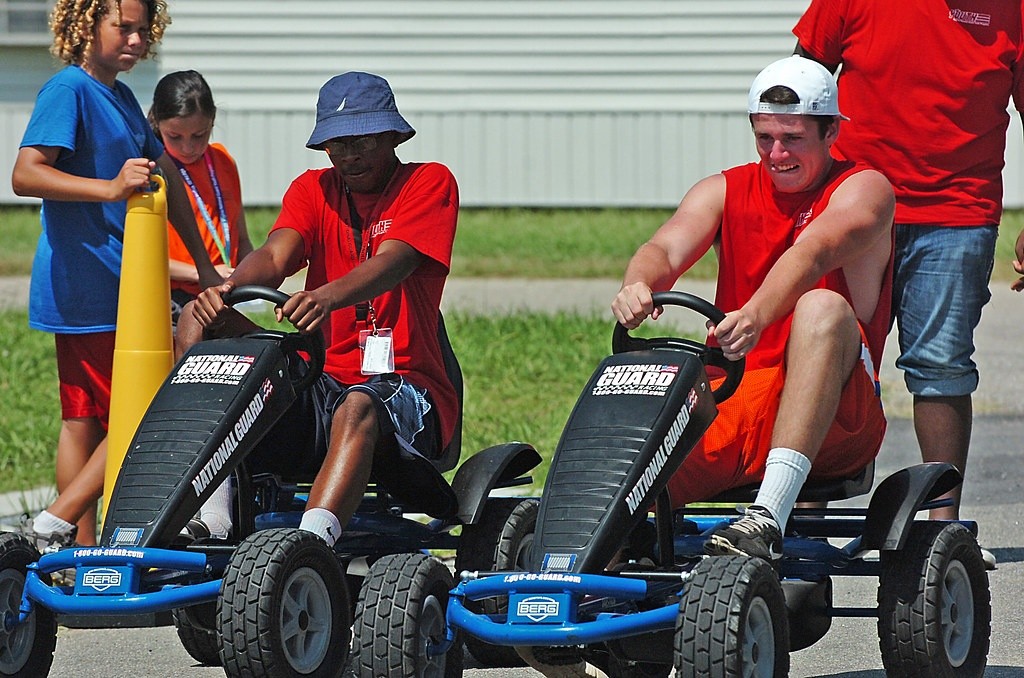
[704, 505, 784, 566]
[975, 536, 997, 571]
[13, 514, 80, 587]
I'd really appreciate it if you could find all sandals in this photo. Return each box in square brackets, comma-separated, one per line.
[174, 518, 234, 551]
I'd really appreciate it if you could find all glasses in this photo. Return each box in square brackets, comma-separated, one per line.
[322, 134, 381, 156]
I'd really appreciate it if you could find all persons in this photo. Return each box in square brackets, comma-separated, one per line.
[792, 0, 1024, 572]
[147, 69, 256, 363]
[11, 0, 171, 550]
[17, 68, 462, 550]
[612, 55, 897, 558]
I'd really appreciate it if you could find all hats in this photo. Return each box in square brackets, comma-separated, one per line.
[747, 54, 852, 121]
[305, 70, 417, 151]
